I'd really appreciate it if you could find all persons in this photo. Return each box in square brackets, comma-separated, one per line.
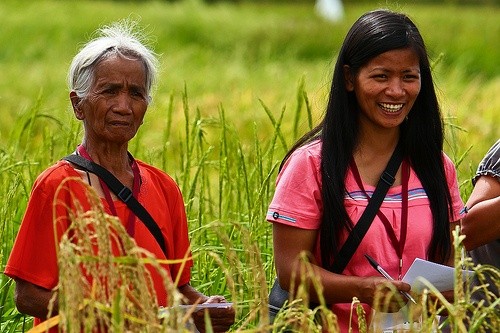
[261, 0, 472, 333]
[441, 137, 500, 333]
[4, 9, 237, 333]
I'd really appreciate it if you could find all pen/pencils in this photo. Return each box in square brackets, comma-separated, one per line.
[364, 252, 419, 306]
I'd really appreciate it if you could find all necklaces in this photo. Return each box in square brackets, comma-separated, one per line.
[75, 149, 93, 191]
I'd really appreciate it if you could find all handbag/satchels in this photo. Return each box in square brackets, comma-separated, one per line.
[267, 276, 297, 317]
